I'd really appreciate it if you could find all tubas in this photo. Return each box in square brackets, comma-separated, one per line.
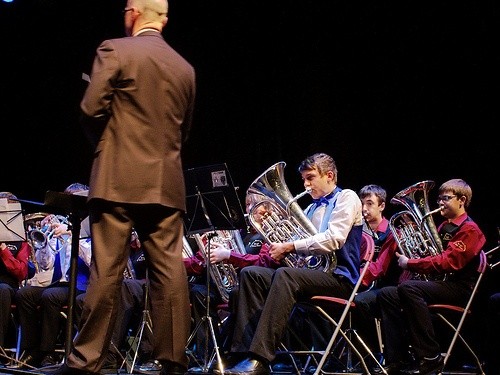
[195, 187, 248, 303]
[388, 180, 447, 283]
[246, 161, 337, 276]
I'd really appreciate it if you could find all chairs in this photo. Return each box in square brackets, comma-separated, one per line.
[273, 232, 389, 375]
[406, 249, 487, 375]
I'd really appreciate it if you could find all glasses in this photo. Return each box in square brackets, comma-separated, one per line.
[438, 195, 457, 201]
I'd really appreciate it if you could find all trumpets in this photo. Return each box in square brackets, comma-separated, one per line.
[25, 213, 72, 256]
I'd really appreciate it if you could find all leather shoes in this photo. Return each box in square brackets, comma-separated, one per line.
[133, 360, 174, 375]
[224, 356, 270, 375]
[163, 364, 186, 374]
[272, 359, 302, 374]
[413, 354, 445, 375]
[36, 364, 99, 375]
[374, 363, 407, 375]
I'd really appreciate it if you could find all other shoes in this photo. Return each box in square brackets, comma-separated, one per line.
[6, 354, 58, 368]
[186, 357, 230, 373]
[305, 363, 336, 375]
[101, 353, 117, 368]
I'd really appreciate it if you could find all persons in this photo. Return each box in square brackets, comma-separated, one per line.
[0, 183, 282, 375]
[309, 184, 416, 375]
[213, 154, 363, 375]
[379, 178, 486, 375]
[45, 0, 197, 375]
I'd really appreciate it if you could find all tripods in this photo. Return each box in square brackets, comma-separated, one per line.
[119, 270, 160, 374]
[178, 187, 248, 374]
